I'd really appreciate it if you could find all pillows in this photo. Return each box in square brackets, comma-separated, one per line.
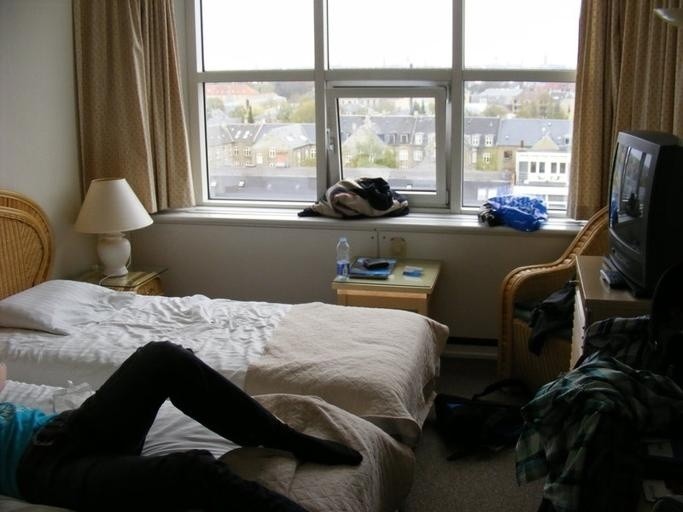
[0, 280, 110, 336]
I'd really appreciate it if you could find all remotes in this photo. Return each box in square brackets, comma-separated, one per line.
[600, 269, 626, 290]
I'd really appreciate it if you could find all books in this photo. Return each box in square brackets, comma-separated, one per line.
[348, 255, 396, 280]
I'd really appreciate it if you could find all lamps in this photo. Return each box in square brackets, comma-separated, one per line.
[74, 178, 153, 278]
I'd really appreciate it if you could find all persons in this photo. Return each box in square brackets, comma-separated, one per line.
[1, 339, 364, 512]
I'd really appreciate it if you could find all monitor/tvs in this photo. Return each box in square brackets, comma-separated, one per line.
[602, 130, 682, 299]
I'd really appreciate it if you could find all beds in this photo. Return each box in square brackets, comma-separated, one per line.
[0, 380, 416, 512]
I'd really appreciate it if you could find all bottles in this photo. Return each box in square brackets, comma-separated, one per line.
[336, 238, 350, 281]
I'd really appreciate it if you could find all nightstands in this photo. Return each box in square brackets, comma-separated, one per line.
[74, 271, 165, 295]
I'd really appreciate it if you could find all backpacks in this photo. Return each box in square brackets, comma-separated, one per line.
[432, 390, 524, 459]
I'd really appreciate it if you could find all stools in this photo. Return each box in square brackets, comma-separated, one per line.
[332, 255, 443, 317]
[0, 188, 449, 438]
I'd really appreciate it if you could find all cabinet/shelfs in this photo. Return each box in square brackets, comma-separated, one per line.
[570, 256, 653, 392]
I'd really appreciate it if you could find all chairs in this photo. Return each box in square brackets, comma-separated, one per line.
[498, 205, 610, 388]
[534, 252, 683, 512]
